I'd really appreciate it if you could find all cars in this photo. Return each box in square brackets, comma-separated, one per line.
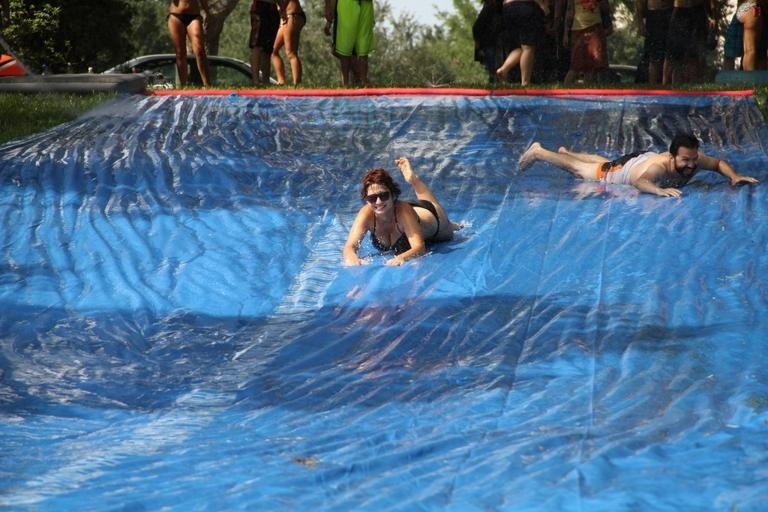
[99, 53, 280, 86]
[578, 64, 638, 84]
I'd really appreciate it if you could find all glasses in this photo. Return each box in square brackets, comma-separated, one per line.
[365, 191, 389, 204]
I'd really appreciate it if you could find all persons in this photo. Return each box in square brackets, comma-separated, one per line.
[165, 1, 211, 90]
[516, 132, 760, 199]
[247, 0, 375, 89]
[472, 1, 767, 88]
[342, 155, 467, 265]
